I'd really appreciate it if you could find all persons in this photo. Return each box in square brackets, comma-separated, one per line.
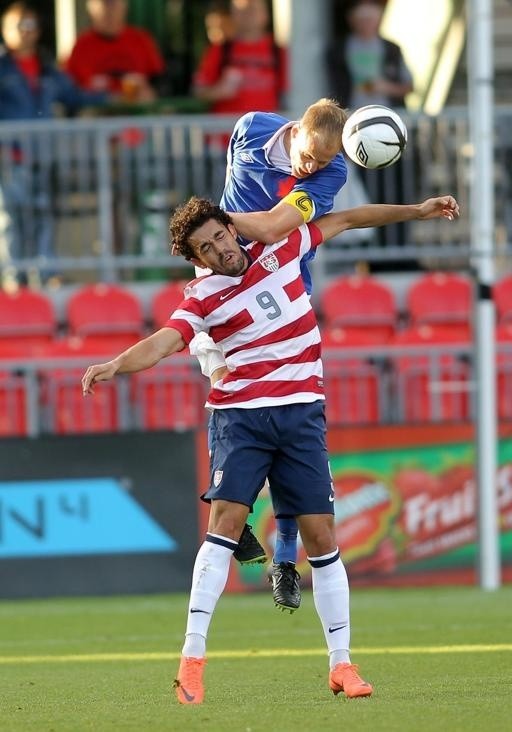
[218, 97, 353, 611]
[0, 2, 108, 344]
[191, 0, 292, 151]
[329, 0, 422, 271]
[79, 193, 462, 706]
[203, 0, 237, 45]
[64, 0, 168, 283]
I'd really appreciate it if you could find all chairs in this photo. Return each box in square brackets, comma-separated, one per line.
[0, 276, 206, 435]
[322, 275, 512, 426]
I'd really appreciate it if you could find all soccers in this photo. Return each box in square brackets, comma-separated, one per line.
[342, 105, 407, 170]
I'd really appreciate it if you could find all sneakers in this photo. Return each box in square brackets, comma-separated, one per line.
[265, 557, 302, 616]
[328, 662, 374, 698]
[176, 651, 208, 706]
[231, 523, 267, 567]
